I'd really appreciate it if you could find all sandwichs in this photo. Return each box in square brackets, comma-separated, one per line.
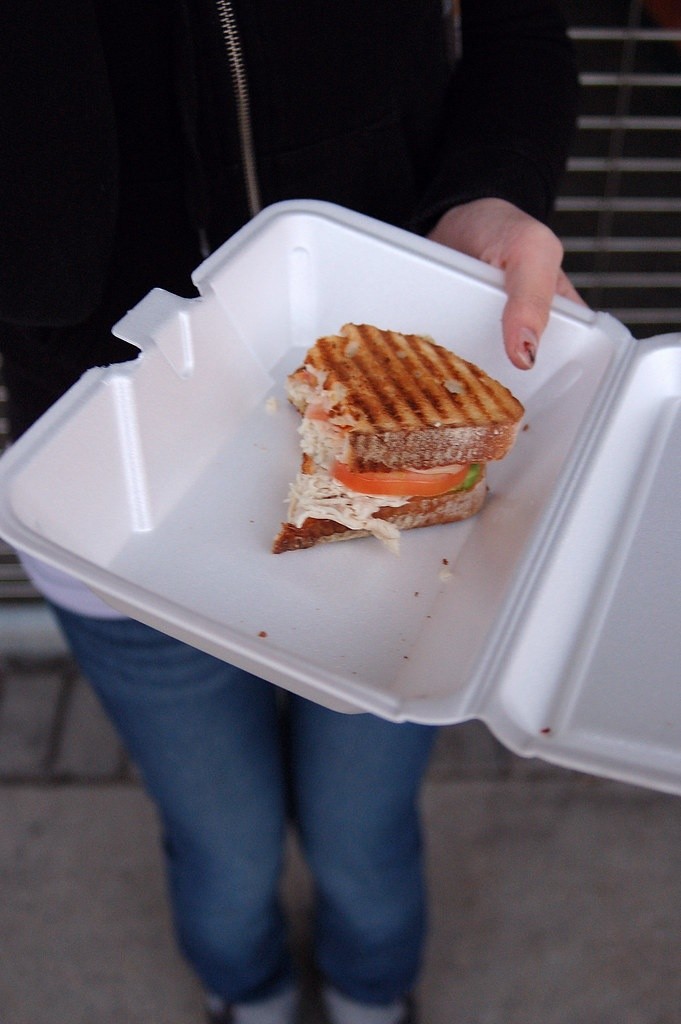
[272, 320, 525, 558]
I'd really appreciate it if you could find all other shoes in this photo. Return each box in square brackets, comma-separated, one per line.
[316, 980, 419, 1024]
[206, 997, 303, 1024]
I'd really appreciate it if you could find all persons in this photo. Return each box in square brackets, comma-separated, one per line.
[0, 0, 593, 1024]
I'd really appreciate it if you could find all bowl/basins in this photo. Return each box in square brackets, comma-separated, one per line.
[0, 199, 681, 799]
